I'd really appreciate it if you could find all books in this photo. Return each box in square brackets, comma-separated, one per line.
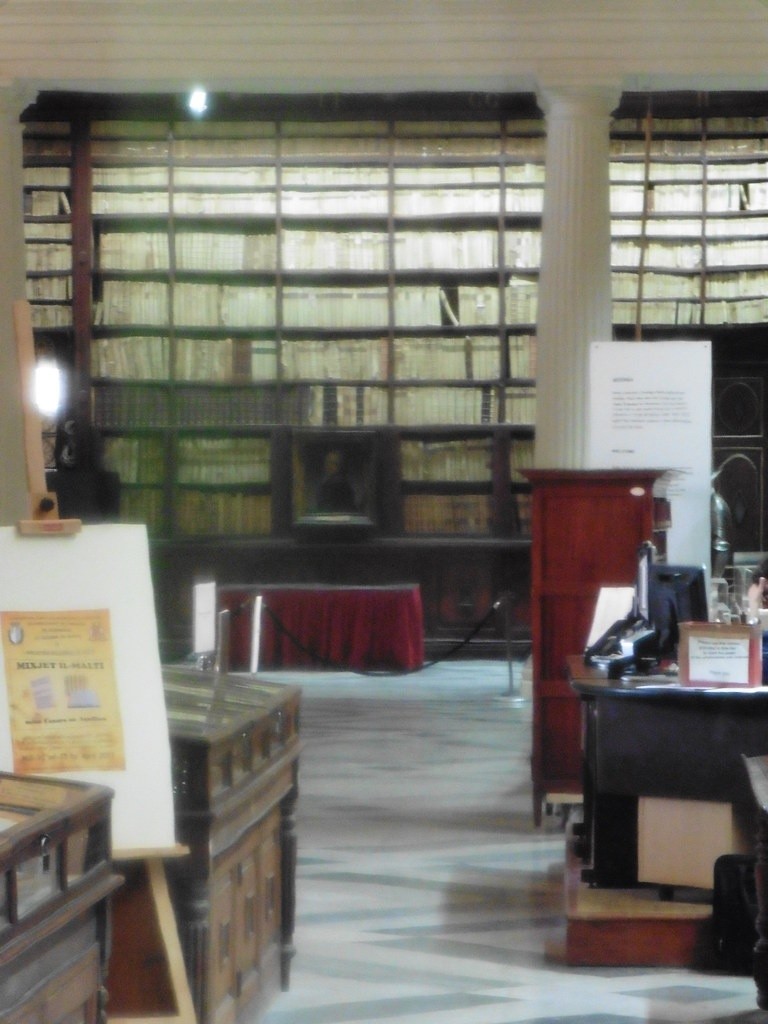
[22, 121, 549, 537]
[609, 116, 768, 325]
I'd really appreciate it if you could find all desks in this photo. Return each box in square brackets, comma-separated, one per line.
[218, 583, 425, 670]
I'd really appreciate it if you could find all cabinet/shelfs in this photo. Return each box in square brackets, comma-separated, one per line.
[569, 678, 767, 900]
[511, 465, 678, 828]
[0, 671, 301, 1023]
[22, 120, 768, 533]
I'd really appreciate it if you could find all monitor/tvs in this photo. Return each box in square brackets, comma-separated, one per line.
[581, 540, 709, 680]
